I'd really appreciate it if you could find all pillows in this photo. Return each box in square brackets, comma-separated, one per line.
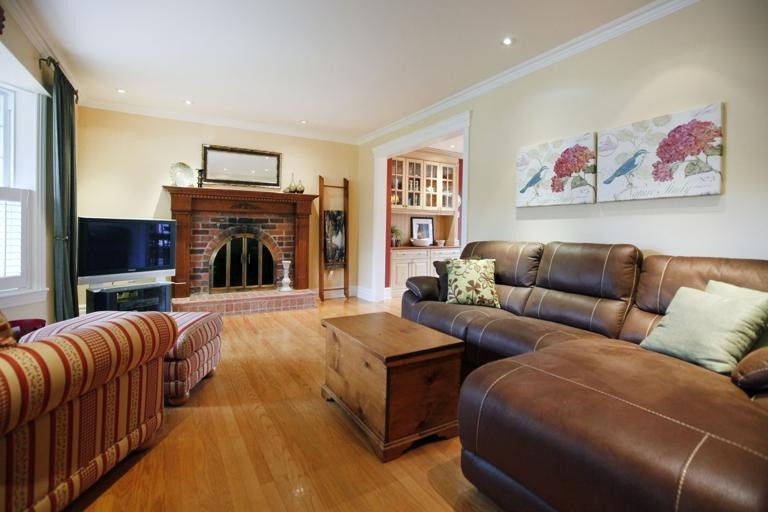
[640, 286, 768, 375]
[445, 257, 501, 308]
[433, 256, 482, 301]
[703, 280, 768, 352]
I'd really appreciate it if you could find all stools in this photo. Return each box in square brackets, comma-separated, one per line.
[18, 311, 223, 406]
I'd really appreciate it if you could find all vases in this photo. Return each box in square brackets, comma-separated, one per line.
[280, 261, 292, 292]
[297, 180, 304, 193]
[289, 172, 296, 192]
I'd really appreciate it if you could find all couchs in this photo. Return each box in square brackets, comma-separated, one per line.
[402, 241, 768, 512]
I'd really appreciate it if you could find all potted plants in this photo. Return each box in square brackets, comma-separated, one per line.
[391, 225, 402, 247]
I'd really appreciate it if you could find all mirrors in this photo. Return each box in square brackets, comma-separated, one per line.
[202, 144, 282, 189]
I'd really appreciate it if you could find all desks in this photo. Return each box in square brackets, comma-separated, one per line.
[86, 283, 172, 313]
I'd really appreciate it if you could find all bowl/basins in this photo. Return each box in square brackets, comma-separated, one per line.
[410, 238, 432, 246]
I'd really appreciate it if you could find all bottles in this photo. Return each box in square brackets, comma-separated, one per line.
[289, 179, 305, 194]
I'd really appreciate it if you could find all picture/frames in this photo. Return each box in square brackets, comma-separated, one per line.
[411, 217, 434, 246]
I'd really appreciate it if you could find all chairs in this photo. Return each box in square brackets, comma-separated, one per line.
[0, 310, 179, 512]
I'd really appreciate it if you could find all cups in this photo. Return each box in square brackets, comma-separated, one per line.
[436, 239, 445, 246]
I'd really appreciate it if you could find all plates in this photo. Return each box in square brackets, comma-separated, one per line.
[170, 162, 194, 186]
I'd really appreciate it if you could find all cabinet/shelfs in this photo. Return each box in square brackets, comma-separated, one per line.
[390, 152, 458, 298]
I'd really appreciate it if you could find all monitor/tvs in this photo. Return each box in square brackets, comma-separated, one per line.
[76, 216, 177, 290]
